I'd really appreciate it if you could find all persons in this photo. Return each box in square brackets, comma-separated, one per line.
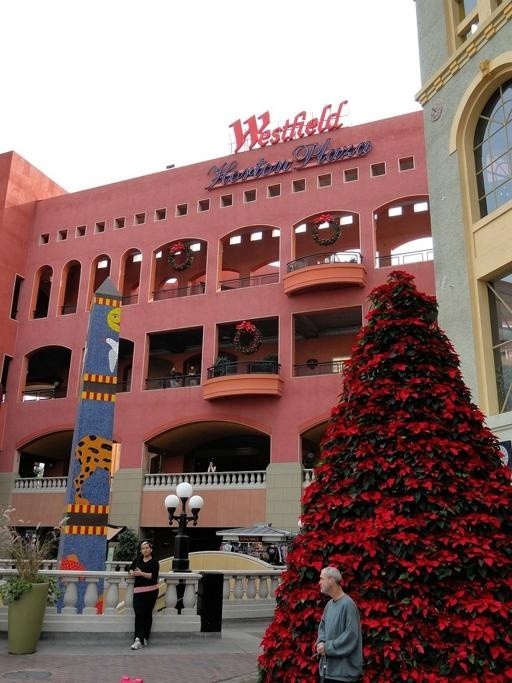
[169, 366, 179, 387]
[207, 457, 217, 473]
[314, 566, 364, 682]
[184, 365, 198, 385]
[128, 540, 159, 649]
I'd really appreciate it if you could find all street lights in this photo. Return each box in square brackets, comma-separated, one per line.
[164, 482, 203, 614]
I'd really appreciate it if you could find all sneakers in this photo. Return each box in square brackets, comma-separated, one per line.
[131, 637, 142, 650]
[141, 639, 150, 646]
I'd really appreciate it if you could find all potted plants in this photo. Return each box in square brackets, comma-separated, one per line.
[0, 504, 73, 657]
